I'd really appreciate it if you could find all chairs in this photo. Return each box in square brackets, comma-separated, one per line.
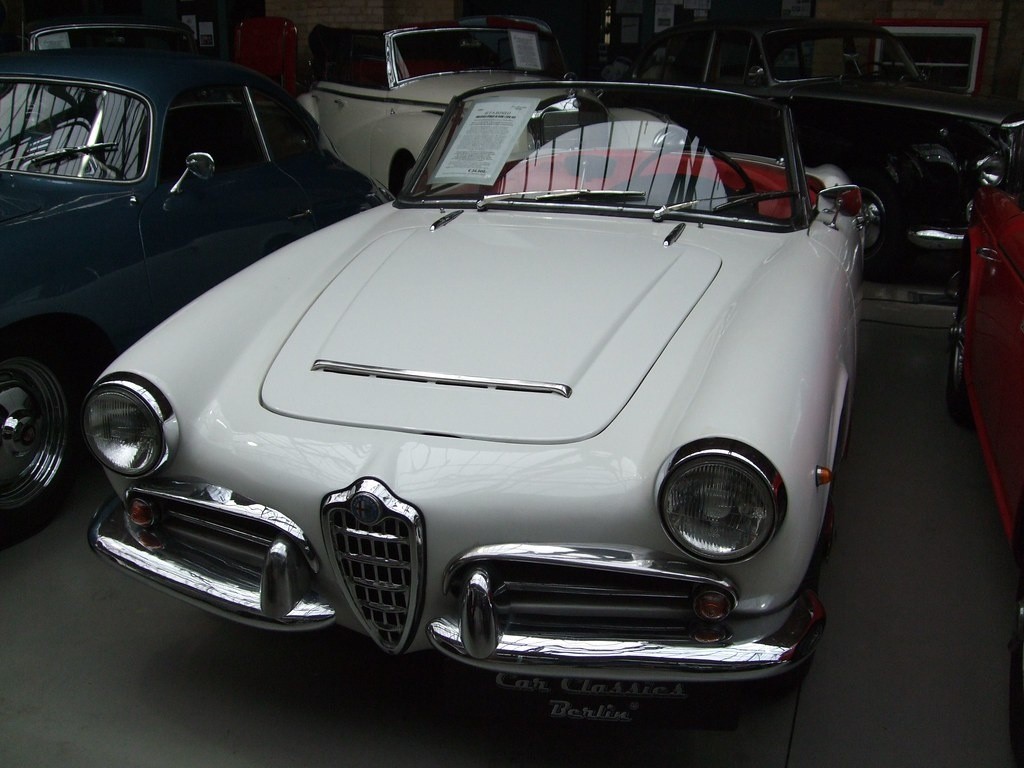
[232, 16, 300, 100]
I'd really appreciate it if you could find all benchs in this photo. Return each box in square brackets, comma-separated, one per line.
[491, 149, 815, 231]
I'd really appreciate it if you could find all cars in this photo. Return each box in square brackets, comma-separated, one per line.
[24, 13, 198, 51]
[295, 15, 691, 196]
[82, 82, 879, 685]
[601, 17, 1023, 281]
[945, 184, 1024, 767]
[0, 49, 386, 542]
